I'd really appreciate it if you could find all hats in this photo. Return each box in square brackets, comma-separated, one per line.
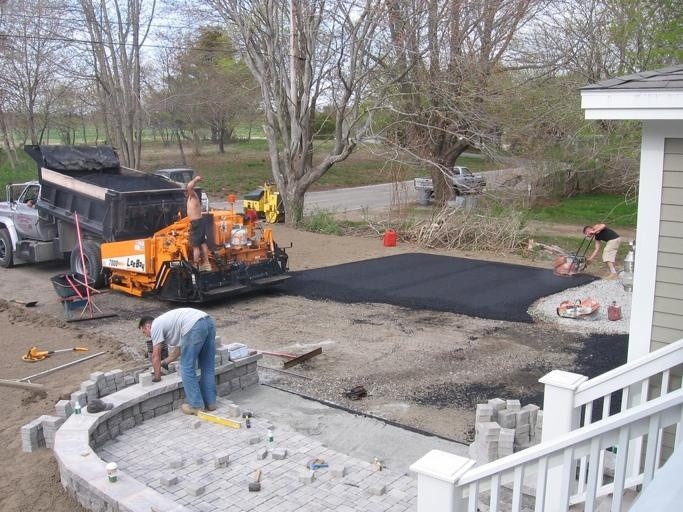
[87, 399, 113, 413]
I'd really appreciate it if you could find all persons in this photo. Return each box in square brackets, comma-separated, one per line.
[26, 187, 39, 206]
[137, 308, 217, 416]
[582, 223, 620, 275]
[182, 175, 212, 273]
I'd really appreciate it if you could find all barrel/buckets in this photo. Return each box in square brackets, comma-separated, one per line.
[384, 229, 396, 245]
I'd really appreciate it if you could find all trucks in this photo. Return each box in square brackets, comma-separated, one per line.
[0, 143, 203, 291]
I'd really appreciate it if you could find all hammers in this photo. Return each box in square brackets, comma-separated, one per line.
[249, 469, 261, 491]
[242, 412, 252, 428]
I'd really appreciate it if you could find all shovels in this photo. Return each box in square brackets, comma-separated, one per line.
[9, 299, 39, 307]
[58, 272, 101, 301]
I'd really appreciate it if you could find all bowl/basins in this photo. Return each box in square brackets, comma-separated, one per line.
[624, 284, 633, 292]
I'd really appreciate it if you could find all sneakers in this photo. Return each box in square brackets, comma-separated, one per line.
[193, 261, 212, 272]
[181, 403, 203, 415]
[207, 404, 216, 411]
[603, 271, 617, 279]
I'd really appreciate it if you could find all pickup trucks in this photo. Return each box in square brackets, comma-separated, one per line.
[414, 166, 486, 199]
